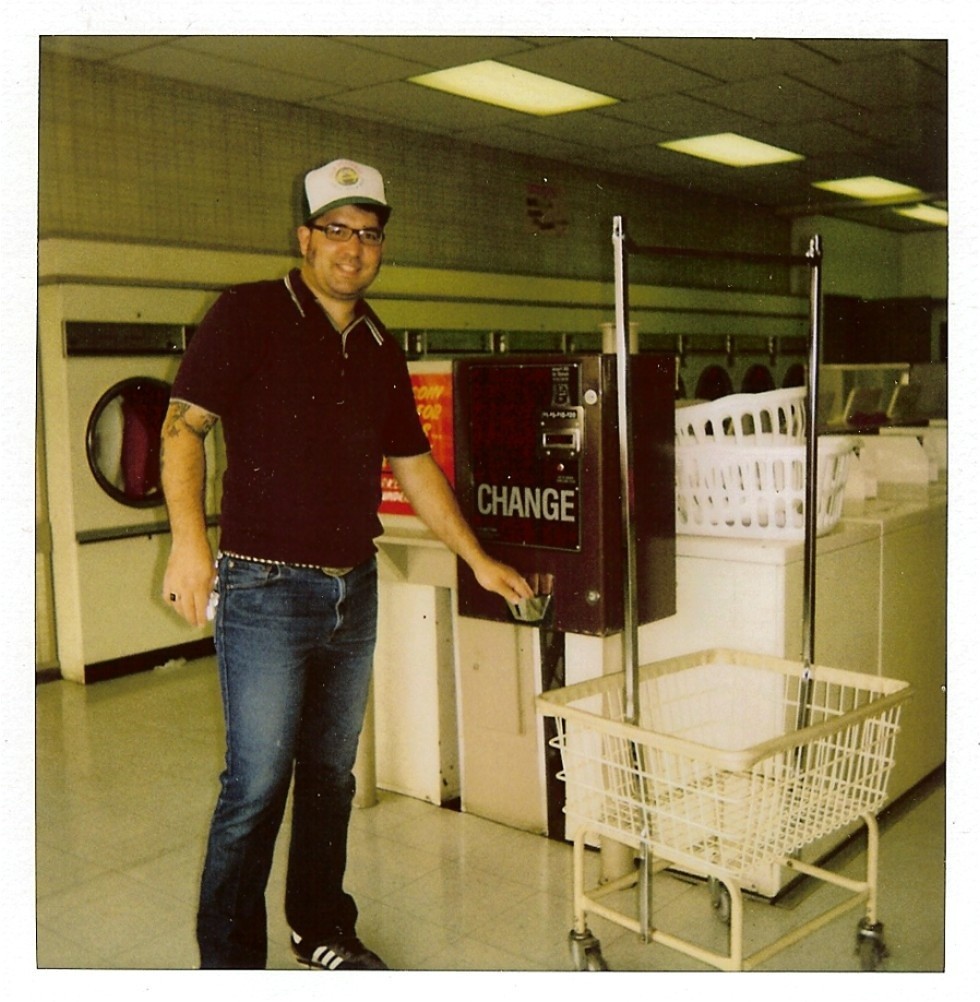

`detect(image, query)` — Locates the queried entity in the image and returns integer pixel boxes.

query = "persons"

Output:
[157,162,539,970]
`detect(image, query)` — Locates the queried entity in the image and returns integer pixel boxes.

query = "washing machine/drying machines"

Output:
[249,254,811,401]
[35,238,248,685]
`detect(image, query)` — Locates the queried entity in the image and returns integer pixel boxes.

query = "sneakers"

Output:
[291,916,390,971]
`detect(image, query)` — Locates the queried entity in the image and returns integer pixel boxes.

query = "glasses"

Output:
[312,222,386,246]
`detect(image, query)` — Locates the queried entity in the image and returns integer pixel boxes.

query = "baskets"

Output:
[675,383,853,541]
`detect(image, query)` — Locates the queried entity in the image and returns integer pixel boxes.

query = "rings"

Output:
[170,591,180,601]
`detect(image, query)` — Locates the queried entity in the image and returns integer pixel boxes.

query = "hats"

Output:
[300,156,394,232]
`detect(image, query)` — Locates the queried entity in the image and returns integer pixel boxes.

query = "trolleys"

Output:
[530,648,914,971]
